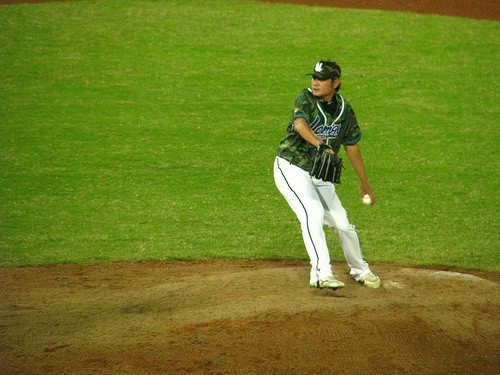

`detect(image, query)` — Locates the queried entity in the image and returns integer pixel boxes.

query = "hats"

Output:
[305,61,341,80]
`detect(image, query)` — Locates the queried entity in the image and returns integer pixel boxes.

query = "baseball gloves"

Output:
[308,143,347,187]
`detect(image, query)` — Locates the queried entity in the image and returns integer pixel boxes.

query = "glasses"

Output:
[313,75,322,80]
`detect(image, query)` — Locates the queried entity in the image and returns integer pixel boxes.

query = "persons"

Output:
[273,61,382,290]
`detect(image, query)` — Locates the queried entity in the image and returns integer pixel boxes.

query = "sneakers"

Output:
[310,278,345,288]
[352,272,381,289]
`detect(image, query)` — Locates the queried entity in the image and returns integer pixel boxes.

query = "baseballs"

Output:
[362,194,372,205]
